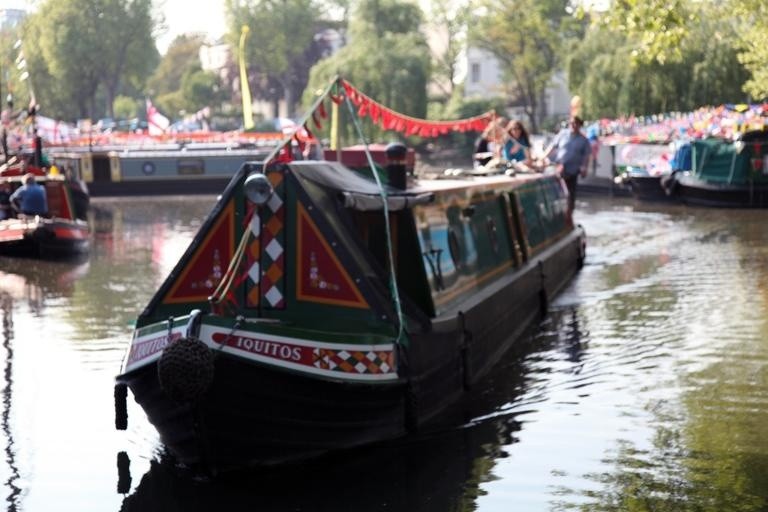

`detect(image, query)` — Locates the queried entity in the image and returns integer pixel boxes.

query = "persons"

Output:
[539,117,591,216]
[499,119,532,168]
[9,173,49,219]
[472,117,507,169]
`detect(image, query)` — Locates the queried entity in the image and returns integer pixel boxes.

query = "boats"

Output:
[0,173,99,258]
[114,78,586,489]
[609,135,768,209]
[14,142,279,198]
